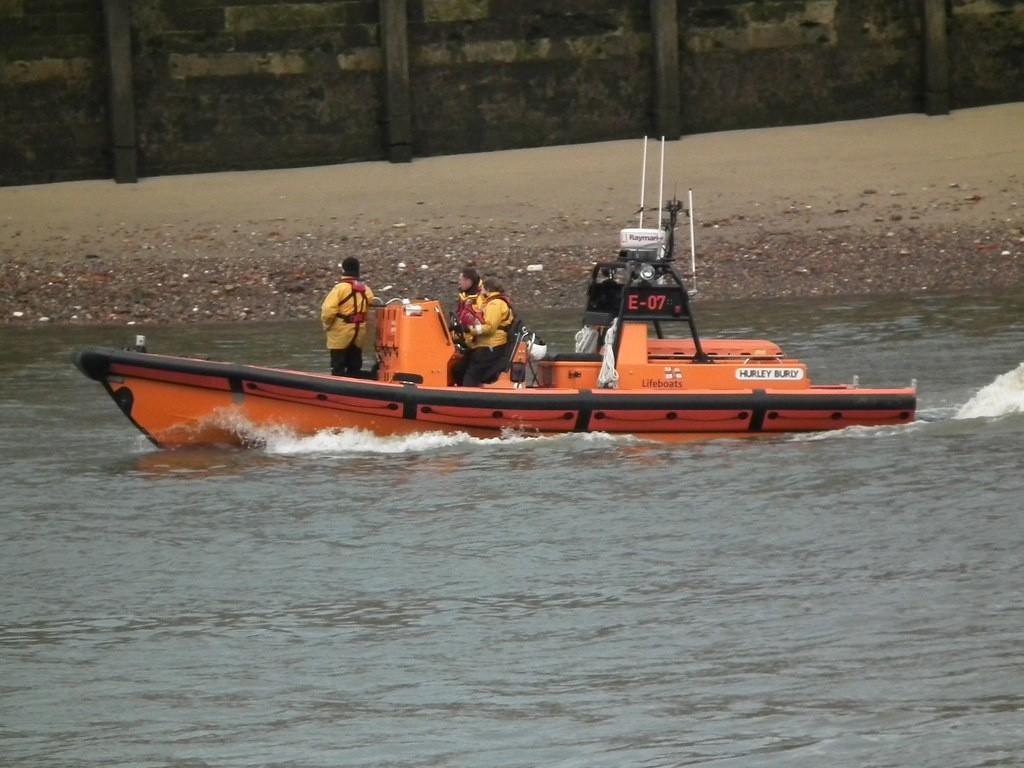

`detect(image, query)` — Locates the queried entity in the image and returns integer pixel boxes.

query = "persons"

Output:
[452,267,516,388]
[319,256,375,380]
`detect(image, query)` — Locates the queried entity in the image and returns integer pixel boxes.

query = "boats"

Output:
[76,134,921,445]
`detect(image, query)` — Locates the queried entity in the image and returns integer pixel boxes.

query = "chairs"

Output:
[479,316,524,383]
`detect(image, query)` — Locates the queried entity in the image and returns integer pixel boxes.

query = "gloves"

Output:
[454,325,468,332]
